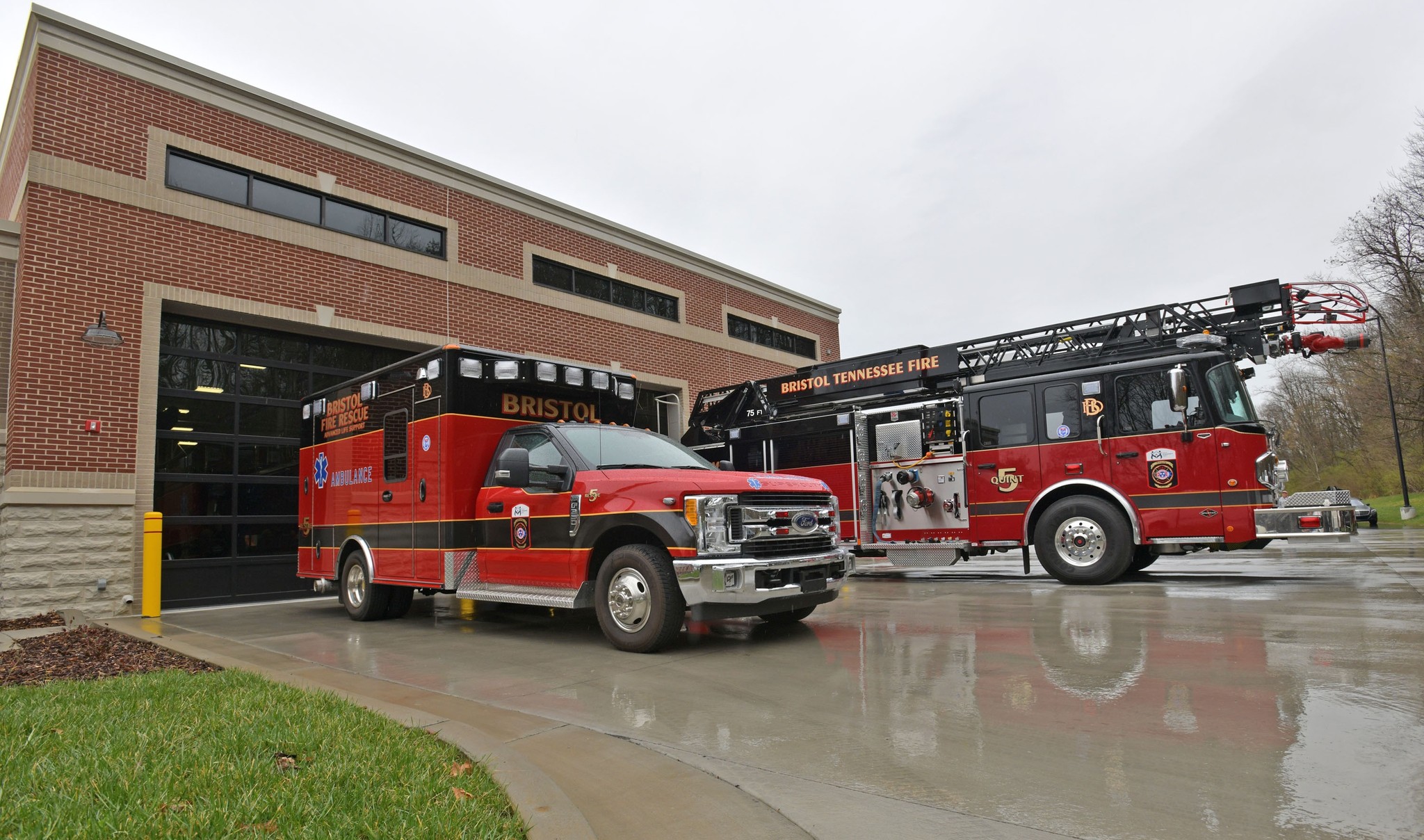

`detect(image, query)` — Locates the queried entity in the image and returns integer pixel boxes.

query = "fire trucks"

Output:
[298,344,856,653]
[678,278,1376,587]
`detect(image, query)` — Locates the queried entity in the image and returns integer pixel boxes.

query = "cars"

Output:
[1351,497,1378,524]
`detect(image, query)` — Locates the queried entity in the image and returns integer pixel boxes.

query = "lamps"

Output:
[81,311,124,350]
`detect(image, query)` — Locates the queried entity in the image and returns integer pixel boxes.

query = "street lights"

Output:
[1350,315,1418,520]
